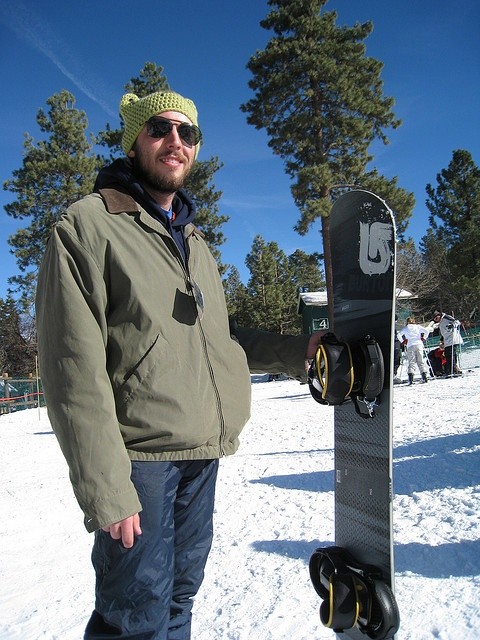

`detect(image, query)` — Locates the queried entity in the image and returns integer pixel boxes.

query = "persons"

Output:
[396,316,429,385]
[37,90,343,640]
[393,314,406,384]
[431,310,462,379]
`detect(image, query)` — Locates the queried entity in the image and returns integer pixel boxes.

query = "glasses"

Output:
[147,116,202,145]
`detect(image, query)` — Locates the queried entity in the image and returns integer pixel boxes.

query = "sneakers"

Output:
[421,378,428,383]
[408,383,415,386]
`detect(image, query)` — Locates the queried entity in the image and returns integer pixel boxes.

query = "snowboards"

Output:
[319,185,399,640]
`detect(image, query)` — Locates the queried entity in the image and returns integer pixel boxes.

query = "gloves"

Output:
[403,339,408,345]
[421,337,425,342]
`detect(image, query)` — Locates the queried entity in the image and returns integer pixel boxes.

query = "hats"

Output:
[120,91,200,161]
[433,310,440,318]
